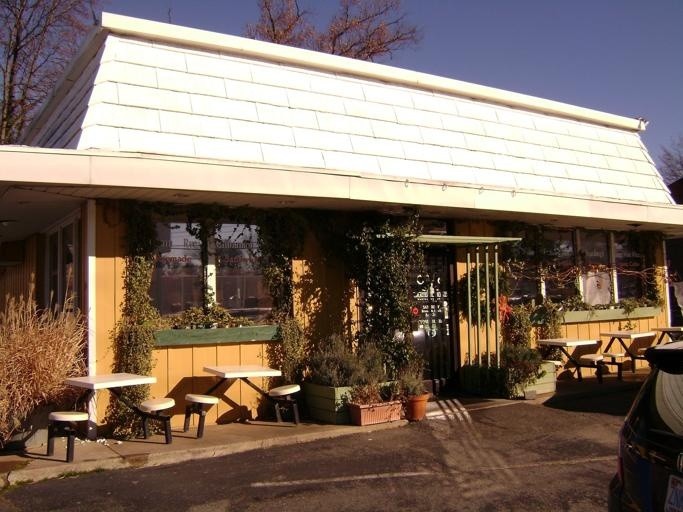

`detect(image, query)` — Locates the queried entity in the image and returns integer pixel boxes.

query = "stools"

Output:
[46,411,90,463]
[538,324,682,387]
[183,394,220,440]
[137,397,177,446]
[267,384,302,426]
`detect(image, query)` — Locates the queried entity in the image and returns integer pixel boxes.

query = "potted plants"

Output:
[400,347,433,422]
[344,375,404,426]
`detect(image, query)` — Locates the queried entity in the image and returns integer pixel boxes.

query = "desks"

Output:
[49,365,176,463]
[182,363,303,440]
